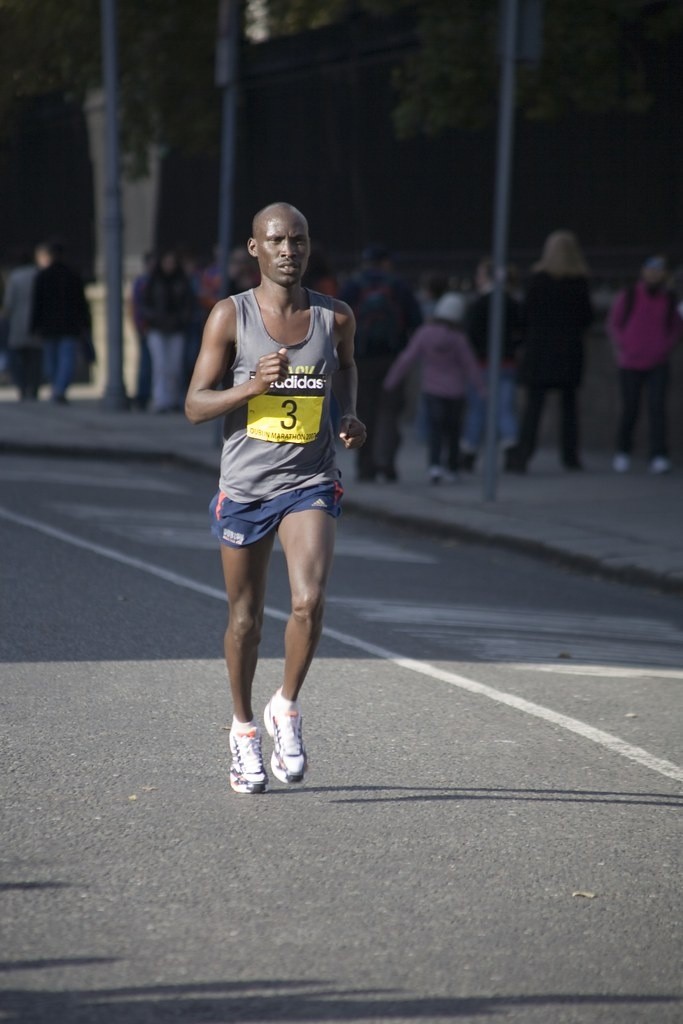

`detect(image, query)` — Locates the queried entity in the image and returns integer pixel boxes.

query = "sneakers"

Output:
[229,724,269,794]
[264,688,307,783]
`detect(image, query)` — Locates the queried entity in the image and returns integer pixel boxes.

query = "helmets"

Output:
[433,293,466,322]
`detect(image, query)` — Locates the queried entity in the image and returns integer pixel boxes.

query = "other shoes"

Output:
[428,466,440,483]
[650,456,670,475]
[444,470,457,483]
[613,453,630,473]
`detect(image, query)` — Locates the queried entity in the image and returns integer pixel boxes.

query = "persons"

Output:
[0,236,90,404]
[185,202,368,794]
[461,252,524,471]
[504,228,597,474]
[134,232,338,415]
[342,243,420,483]
[605,249,683,474]
[385,287,489,485]
